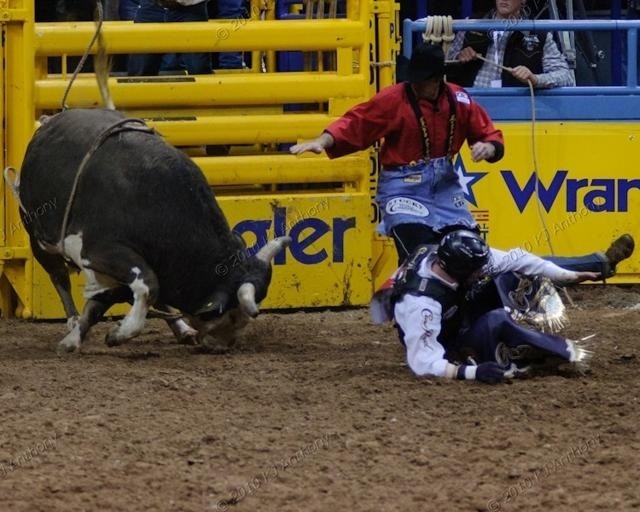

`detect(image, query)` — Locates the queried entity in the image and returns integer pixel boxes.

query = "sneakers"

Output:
[605,234,634,277]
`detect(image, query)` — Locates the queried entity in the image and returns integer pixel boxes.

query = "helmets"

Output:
[437,229,489,284]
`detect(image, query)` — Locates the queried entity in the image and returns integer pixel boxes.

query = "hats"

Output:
[397,44,469,81]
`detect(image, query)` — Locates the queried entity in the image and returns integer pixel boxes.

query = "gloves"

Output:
[457,362,509,386]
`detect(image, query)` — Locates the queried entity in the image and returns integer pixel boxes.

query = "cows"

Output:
[18,107,293,360]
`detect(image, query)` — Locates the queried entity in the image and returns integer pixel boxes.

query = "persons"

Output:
[126,1,232,157]
[290,39,505,266]
[443,3,575,93]
[360,226,636,387]
[219,0,304,151]
